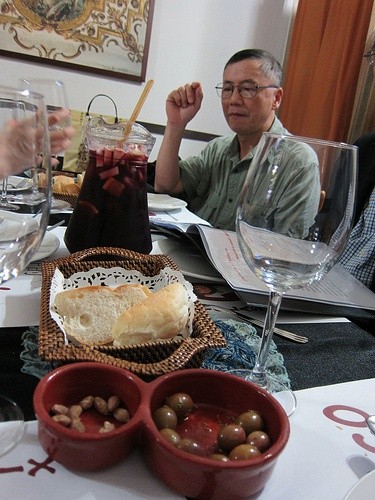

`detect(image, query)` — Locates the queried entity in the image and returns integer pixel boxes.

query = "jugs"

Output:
[64,116,156,255]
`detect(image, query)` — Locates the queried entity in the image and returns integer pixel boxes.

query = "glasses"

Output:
[215,80,280,98]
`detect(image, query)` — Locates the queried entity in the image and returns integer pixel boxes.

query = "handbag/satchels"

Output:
[61,94,129,173]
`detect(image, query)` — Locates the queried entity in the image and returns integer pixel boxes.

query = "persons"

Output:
[0,105,76,182]
[193,282,233,300]
[333,187,375,291]
[154,48,322,240]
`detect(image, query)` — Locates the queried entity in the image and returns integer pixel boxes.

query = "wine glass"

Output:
[224,132,358,417]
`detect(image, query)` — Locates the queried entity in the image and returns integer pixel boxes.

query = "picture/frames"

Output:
[0,0,155,83]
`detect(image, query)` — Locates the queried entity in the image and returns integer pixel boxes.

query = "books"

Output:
[149,219,375,317]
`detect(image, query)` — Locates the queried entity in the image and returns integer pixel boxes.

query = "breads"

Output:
[54,283,151,345]
[112,282,190,346]
[37,172,80,195]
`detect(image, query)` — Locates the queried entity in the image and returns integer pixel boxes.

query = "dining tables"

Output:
[1,173,375,500]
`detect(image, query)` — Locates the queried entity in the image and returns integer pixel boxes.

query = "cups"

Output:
[1,87,53,285]
[14,78,69,156]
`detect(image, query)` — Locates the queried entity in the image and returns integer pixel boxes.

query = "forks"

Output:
[1,178,29,189]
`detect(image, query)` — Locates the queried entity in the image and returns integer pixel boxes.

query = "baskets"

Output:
[37,246,228,375]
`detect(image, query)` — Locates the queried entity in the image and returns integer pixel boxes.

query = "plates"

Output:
[148,192,188,211]
[150,237,227,283]
[0,175,34,192]
[343,471,375,500]
[31,230,60,262]
